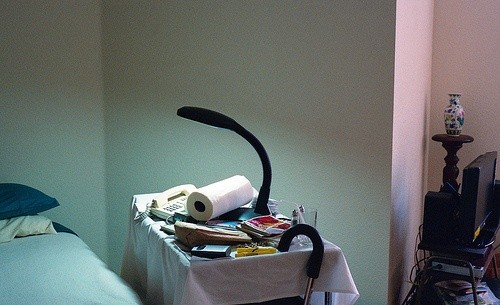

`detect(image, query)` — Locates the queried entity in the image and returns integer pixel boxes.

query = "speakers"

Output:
[492,180,500,226]
[422,189,460,245]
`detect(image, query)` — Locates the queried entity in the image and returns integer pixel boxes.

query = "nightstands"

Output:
[118,192,360,305]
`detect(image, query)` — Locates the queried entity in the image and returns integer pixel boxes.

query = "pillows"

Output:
[0,183,60,220]
[0,213,57,243]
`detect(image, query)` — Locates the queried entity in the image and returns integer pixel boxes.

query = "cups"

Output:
[291,208,318,247]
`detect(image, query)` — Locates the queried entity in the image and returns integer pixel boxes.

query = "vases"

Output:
[444,94,464,136]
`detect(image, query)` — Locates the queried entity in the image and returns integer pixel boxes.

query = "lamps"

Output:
[177,107,272,221]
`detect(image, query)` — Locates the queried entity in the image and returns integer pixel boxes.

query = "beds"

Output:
[0,215,142,305]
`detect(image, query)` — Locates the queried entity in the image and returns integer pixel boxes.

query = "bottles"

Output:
[444,93,464,136]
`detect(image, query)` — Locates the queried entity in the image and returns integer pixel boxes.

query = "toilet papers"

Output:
[186,174,254,222]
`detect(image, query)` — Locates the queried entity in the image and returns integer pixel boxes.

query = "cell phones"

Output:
[191,244,231,257]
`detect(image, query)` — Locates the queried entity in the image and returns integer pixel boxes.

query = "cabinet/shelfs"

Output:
[415,242,499,305]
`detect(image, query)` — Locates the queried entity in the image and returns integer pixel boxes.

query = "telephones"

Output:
[150,183,197,222]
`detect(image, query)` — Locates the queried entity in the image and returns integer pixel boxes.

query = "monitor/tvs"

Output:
[460,149,498,248]
